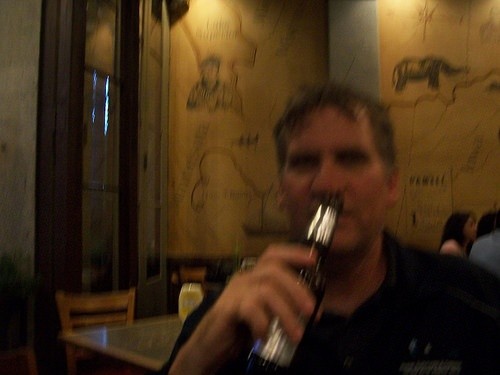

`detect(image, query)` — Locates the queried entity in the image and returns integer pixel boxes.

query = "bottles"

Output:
[244,194,343,375]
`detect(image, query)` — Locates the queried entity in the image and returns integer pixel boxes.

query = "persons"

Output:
[438,211,500,277]
[160,88,500,375]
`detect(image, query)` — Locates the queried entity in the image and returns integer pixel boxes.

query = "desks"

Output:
[58,315,186,375]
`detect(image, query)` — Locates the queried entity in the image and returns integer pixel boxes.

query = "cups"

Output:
[178,283,205,323]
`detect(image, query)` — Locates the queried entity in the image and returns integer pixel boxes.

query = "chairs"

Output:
[58,286,135,375]
